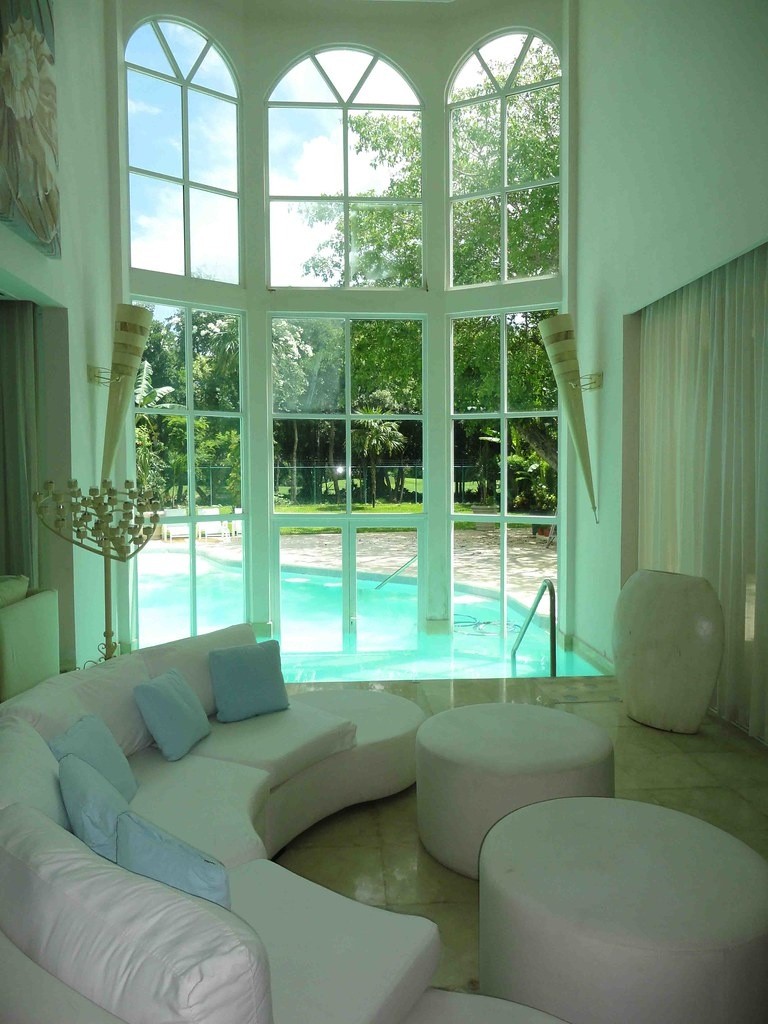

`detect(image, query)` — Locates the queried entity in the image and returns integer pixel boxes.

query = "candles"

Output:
[34,479,160,555]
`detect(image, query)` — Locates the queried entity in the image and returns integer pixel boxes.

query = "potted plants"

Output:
[471,444,499,531]
[516,469,556,535]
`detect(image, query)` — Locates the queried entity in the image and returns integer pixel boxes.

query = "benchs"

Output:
[162,508,242,542]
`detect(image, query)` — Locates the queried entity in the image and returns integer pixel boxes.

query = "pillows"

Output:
[209,640,290,722]
[56,754,129,862]
[47,713,138,804]
[114,811,232,911]
[132,665,211,762]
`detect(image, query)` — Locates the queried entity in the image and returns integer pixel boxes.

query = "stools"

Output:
[479,797,768,1024]
[417,702,616,881]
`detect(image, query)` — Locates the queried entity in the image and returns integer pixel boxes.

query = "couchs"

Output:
[0,624,440,1024]
[0,573,59,702]
